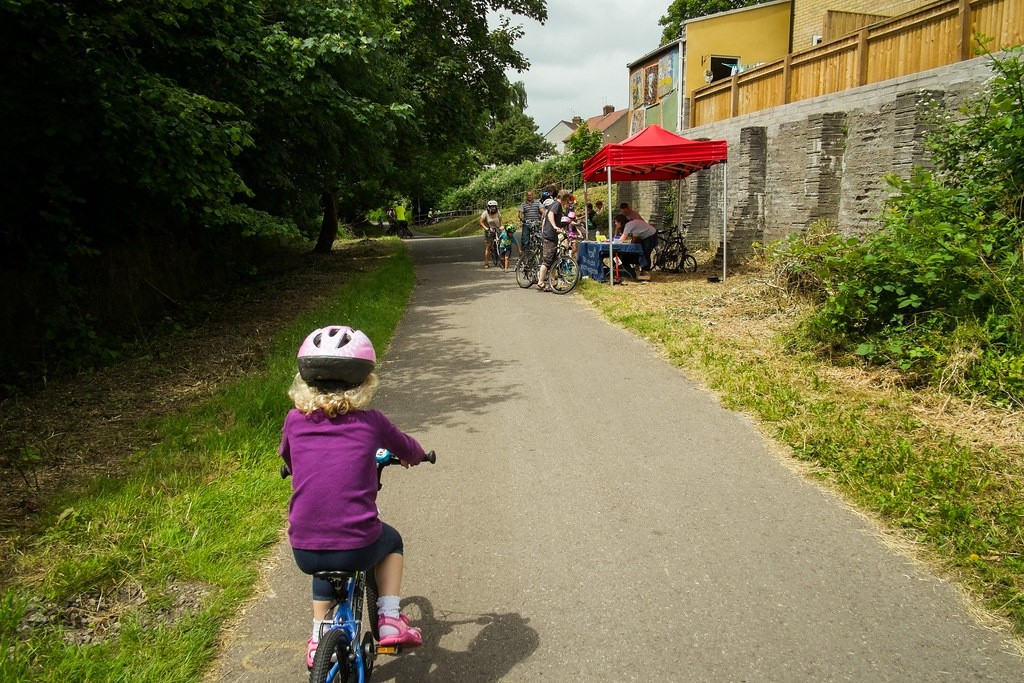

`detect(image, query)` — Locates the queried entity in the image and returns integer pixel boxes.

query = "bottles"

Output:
[596,230,600,238]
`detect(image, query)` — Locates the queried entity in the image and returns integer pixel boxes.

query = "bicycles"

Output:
[278,447,437,683]
[514,217,582,295]
[650,224,697,273]
[377,222,414,238]
[485,228,504,267]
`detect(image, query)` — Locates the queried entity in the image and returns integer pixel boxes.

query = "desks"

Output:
[580,241,642,283]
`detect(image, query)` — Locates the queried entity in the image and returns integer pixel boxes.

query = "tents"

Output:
[583,124,728,287]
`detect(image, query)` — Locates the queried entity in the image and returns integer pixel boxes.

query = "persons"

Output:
[480,189,657,292]
[377,216,383,224]
[278,325,424,668]
[426,208,433,226]
[388,208,395,217]
[394,202,406,222]
[434,210,442,222]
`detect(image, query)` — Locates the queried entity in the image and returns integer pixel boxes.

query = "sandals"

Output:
[377,613,423,646]
[553,284,565,292]
[536,283,552,292]
[306,636,320,672]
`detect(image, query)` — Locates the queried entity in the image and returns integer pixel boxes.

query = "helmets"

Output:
[504,224,517,233]
[487,201,498,214]
[296,325,376,385]
[568,212,576,219]
[542,192,551,202]
[560,216,571,223]
